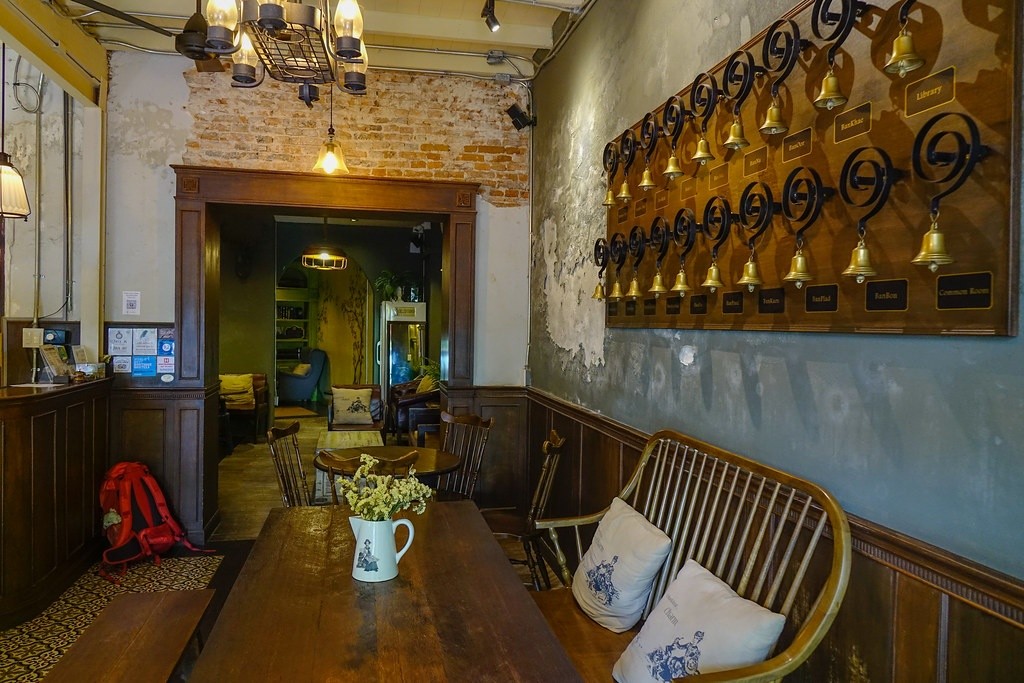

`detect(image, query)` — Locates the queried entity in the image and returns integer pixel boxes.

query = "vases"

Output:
[349,517,415,582]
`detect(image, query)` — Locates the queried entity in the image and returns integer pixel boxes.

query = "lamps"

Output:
[301,216,349,271]
[505,103,538,131]
[481,0,500,33]
[207,0,373,111]
[313,23,348,177]
[0,151,31,222]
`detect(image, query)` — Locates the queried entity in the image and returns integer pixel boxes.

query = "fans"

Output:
[70,0,225,72]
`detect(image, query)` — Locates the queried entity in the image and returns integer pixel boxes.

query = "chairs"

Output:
[482,430,567,592]
[391,380,438,446]
[313,446,461,507]
[409,407,441,448]
[327,384,390,446]
[432,410,494,504]
[320,450,419,505]
[223,373,268,443]
[267,420,311,508]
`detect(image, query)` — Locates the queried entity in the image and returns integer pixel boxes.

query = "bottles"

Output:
[411,282,418,302]
[280,306,293,319]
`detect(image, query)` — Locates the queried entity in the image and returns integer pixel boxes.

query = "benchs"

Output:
[41,588,224,683]
[527,427,851,683]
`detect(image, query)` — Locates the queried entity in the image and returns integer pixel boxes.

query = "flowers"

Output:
[336,453,436,521]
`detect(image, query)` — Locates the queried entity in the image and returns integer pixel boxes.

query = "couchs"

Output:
[277,347,324,400]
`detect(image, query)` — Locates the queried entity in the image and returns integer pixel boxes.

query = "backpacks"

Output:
[98,461,217,586]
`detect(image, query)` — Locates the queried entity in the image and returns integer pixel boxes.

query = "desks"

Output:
[187,502,589,683]
[315,431,383,507]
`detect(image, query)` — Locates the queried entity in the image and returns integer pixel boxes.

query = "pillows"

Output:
[612,559,786,683]
[416,374,436,394]
[293,364,311,376]
[331,387,373,424]
[219,374,255,410]
[572,498,671,633]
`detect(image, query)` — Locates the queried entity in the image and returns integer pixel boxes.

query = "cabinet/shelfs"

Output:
[0,375,113,632]
[276,287,319,403]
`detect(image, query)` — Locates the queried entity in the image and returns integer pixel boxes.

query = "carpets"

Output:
[0,539,256,683]
[275,405,323,419]
